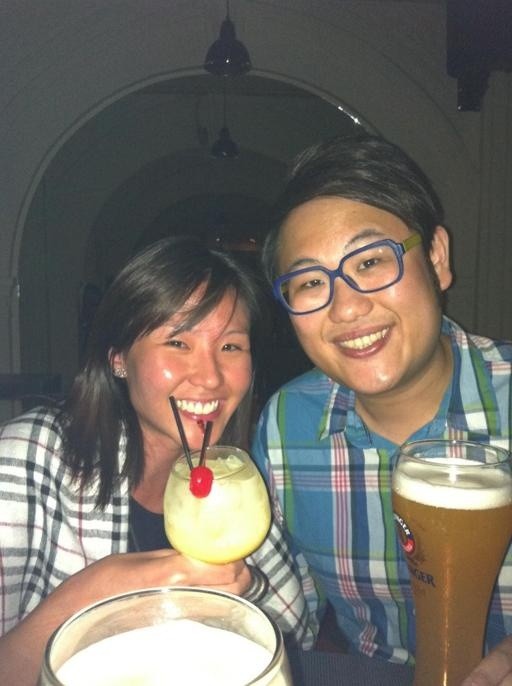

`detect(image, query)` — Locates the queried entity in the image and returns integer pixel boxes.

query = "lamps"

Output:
[204,0,253,80]
[209,92,241,162]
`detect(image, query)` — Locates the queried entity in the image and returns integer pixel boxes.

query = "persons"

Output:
[0,229,328,686]
[241,129,512,686]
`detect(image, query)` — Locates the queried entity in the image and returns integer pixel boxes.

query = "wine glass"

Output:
[162,445,271,605]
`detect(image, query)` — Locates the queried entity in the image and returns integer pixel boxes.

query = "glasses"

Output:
[274,233,421,315]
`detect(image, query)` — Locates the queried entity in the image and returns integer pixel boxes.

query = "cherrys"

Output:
[190,466,212,498]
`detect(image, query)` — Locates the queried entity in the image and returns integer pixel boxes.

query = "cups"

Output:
[388,437,512,686]
[37,583,295,686]
